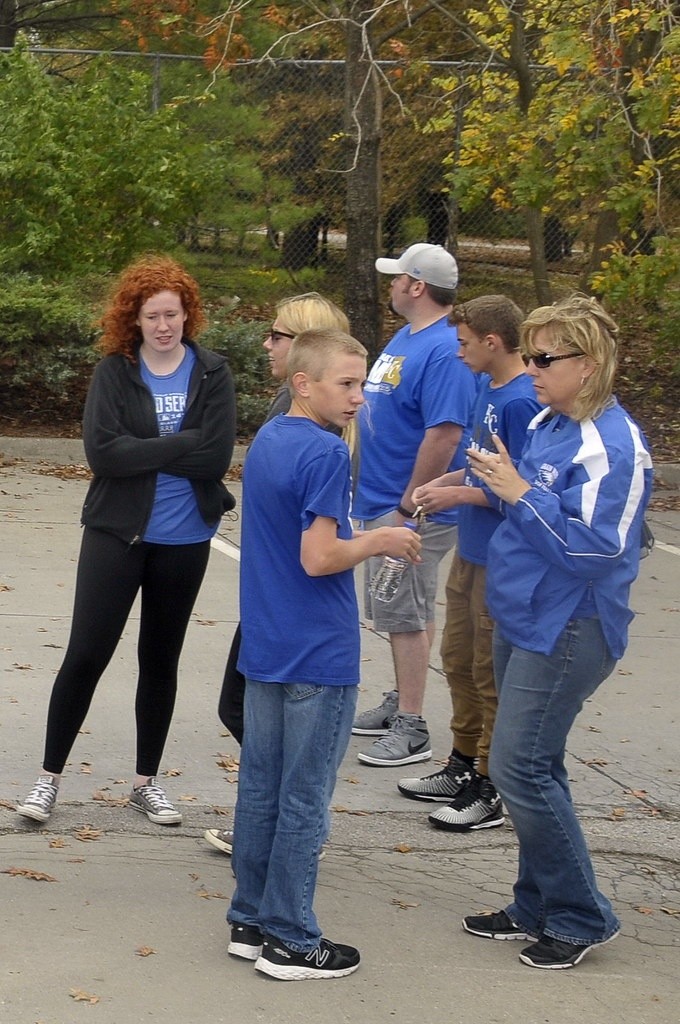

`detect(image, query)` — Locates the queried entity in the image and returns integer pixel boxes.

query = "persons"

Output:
[16,259,235,823]
[543,203,572,271]
[460,298,654,969]
[621,211,673,276]
[351,244,478,766]
[397,294,546,833]
[205,293,422,980]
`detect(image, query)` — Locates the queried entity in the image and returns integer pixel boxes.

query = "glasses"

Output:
[270,328,294,341]
[521,352,586,368]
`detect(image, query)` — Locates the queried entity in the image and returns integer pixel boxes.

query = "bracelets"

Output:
[397,504,420,520]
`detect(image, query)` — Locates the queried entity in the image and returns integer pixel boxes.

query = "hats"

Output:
[375,243,458,289]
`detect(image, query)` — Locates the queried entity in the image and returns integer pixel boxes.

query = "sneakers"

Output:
[317,846,326,860]
[462,910,539,943]
[227,921,263,960]
[357,711,432,766]
[204,828,234,854]
[19,774,59,822]
[428,772,505,832]
[128,776,183,824]
[351,690,399,735]
[398,755,475,802]
[519,922,623,970]
[254,934,360,980]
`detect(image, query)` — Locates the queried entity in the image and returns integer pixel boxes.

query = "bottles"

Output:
[368,520,417,604]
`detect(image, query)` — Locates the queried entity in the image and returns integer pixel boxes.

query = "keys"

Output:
[412,505,423,517]
[419,512,425,523]
[411,503,429,529]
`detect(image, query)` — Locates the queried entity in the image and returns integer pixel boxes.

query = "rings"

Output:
[485,469,493,475]
